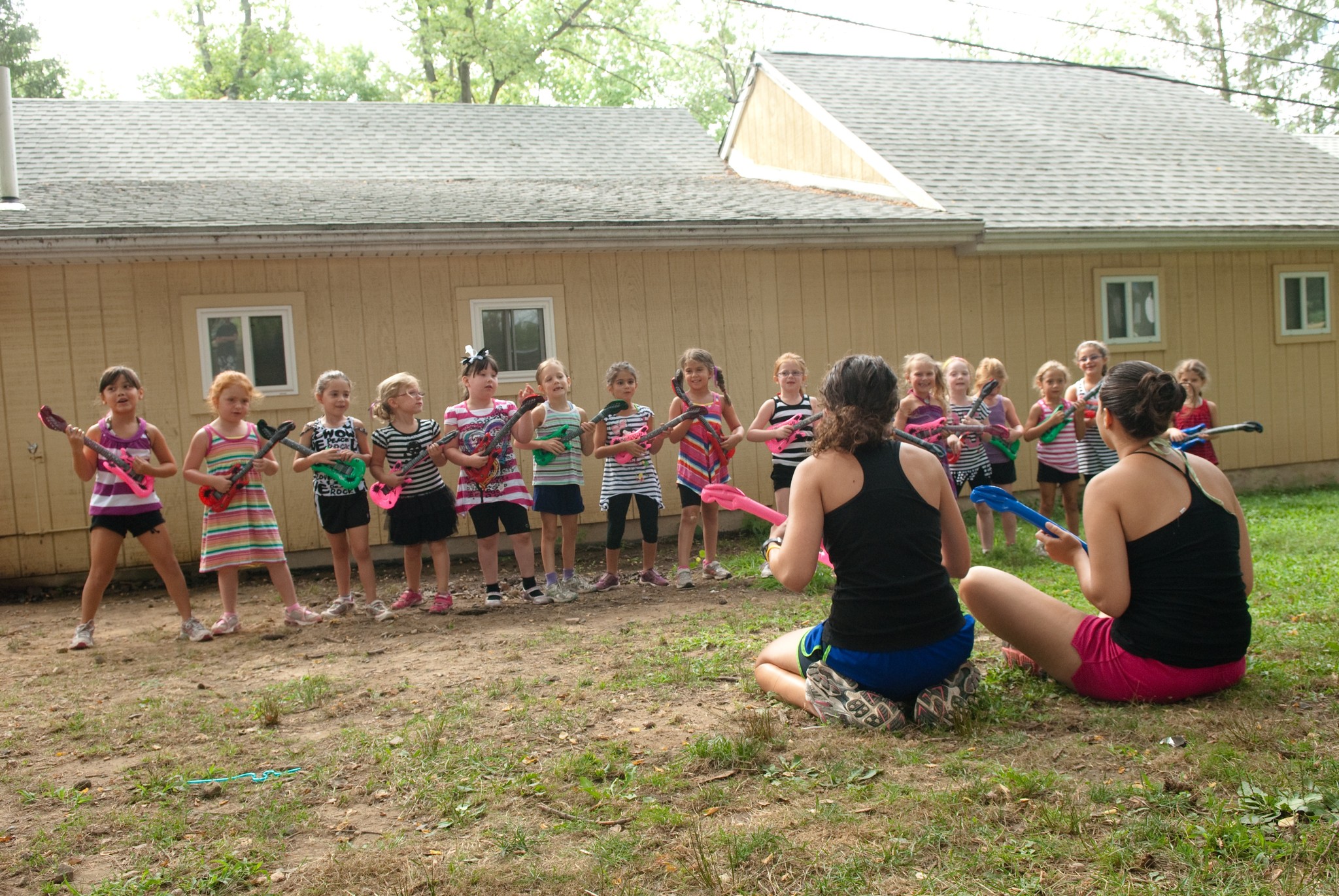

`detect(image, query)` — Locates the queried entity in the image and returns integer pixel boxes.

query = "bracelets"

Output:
[763,545,783,563]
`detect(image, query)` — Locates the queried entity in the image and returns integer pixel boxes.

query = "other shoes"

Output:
[1035,540,1050,557]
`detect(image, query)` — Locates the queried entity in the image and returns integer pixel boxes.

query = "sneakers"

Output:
[674,565,695,588]
[283,604,322,626]
[522,585,551,604]
[701,559,732,580]
[390,586,424,610]
[592,571,620,592]
[68,623,96,649]
[364,601,395,622]
[544,581,578,603]
[319,594,359,620]
[803,659,908,734]
[210,611,241,635]
[561,575,597,594]
[428,591,453,614]
[484,591,503,608]
[912,659,981,731]
[639,568,670,587]
[1001,637,1048,678]
[760,561,774,578]
[178,616,213,642]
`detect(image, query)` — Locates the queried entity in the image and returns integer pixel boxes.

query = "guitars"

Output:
[968,483,1094,557]
[946,379,999,463]
[902,418,1011,443]
[531,399,629,467]
[1169,421,1264,449]
[670,369,736,468]
[610,405,707,465]
[464,394,545,483]
[256,418,366,490]
[1034,376,1104,444]
[368,430,459,509]
[699,483,835,574]
[988,430,1021,458]
[197,421,296,514]
[763,406,828,455]
[36,404,155,498]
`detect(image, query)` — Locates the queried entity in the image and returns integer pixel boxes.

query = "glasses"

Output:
[1077,354,1104,361]
[393,389,425,399]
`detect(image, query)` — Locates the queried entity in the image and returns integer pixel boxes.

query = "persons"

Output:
[590,360,675,593]
[1160,360,1223,470]
[181,368,325,637]
[887,352,961,502]
[1021,357,1087,557]
[953,358,1255,706]
[751,354,982,729]
[213,318,238,371]
[746,352,834,581]
[940,356,997,554]
[62,363,214,649]
[668,348,746,591]
[1066,336,1119,489]
[514,356,599,605]
[438,345,550,608]
[973,358,1026,551]
[370,372,459,616]
[292,368,399,626]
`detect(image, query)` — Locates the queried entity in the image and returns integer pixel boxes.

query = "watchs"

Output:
[756,537,784,561]
